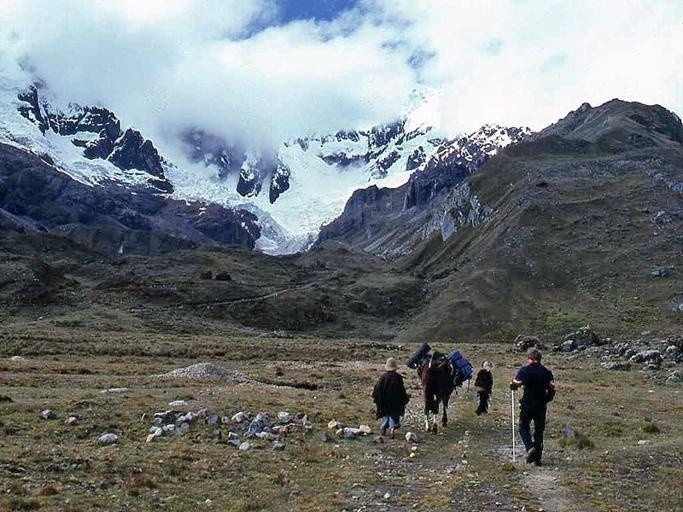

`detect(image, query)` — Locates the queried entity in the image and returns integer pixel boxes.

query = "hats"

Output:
[384,357,399,372]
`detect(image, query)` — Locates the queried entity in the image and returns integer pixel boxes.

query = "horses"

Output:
[417,358,456,434]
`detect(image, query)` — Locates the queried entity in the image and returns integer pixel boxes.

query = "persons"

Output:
[509,347,556,466]
[474,360,493,416]
[371,357,409,439]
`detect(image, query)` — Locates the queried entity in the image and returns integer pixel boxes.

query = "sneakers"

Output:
[527,448,537,462]
